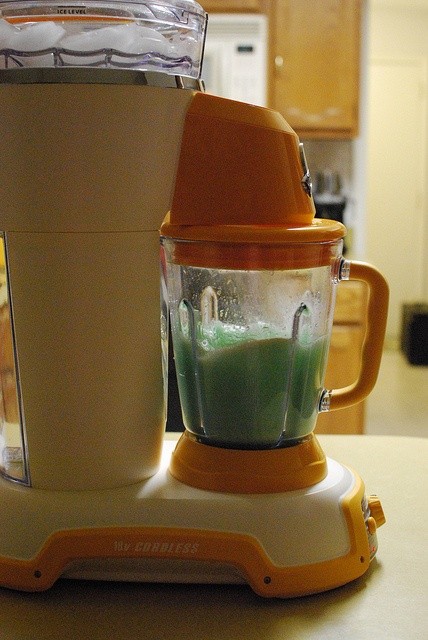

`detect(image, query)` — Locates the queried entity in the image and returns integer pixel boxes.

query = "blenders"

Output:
[0,1,389,598]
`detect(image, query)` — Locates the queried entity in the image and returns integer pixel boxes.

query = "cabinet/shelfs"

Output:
[266,0,362,140]
